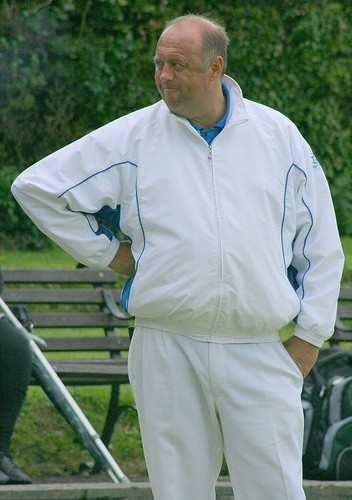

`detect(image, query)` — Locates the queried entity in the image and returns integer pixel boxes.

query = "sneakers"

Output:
[1,456,33,483]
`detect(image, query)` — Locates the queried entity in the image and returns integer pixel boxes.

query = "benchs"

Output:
[0,268,314,475]
[310,286,352,481]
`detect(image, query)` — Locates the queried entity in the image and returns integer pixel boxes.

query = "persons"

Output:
[9,15,347,500]
